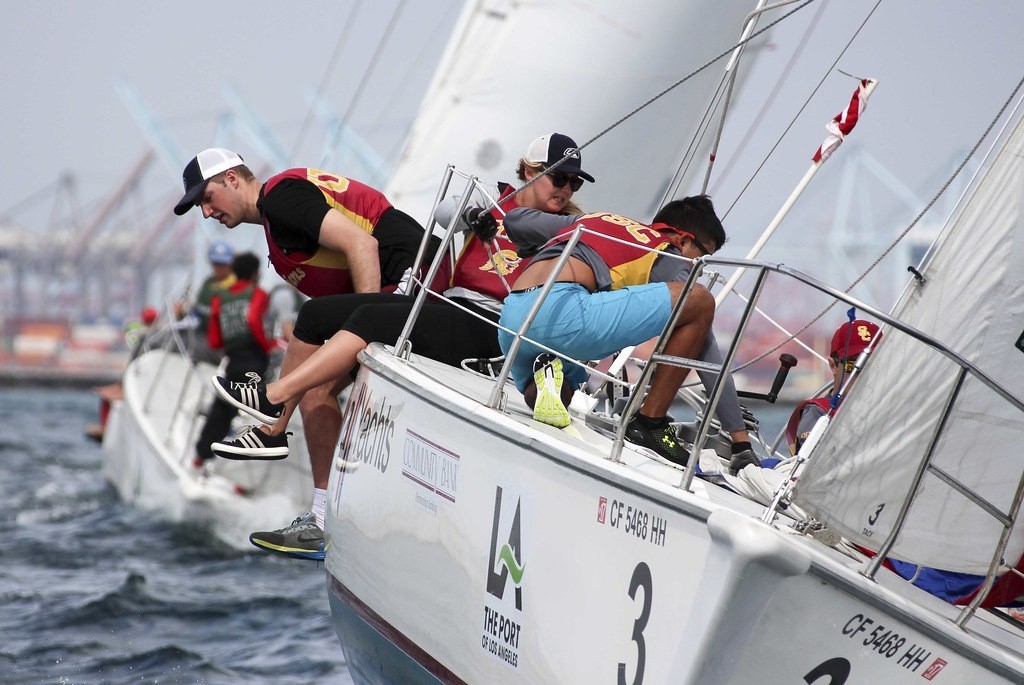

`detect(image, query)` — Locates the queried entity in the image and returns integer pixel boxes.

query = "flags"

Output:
[812,79,868,163]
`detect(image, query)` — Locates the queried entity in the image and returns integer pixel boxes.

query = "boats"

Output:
[97,265,327,561]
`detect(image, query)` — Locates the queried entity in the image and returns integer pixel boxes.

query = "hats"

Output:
[141,308,157,324]
[173,149,244,216]
[830,320,883,360]
[209,241,233,264]
[527,133,595,183]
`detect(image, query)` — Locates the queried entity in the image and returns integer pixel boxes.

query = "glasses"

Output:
[833,357,866,374]
[537,166,584,192]
[691,237,712,266]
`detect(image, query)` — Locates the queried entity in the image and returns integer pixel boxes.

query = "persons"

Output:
[784,320,883,458]
[211,132,595,462]
[85,240,307,472]
[498,194,760,477]
[174,148,457,563]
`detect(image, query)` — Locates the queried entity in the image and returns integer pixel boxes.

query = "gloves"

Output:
[469,207,498,242]
[729,449,763,476]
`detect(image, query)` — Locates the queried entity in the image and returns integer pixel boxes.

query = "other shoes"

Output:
[191,456,208,475]
[235,478,253,500]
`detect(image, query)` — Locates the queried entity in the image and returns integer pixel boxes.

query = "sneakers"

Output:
[623,410,690,470]
[210,423,294,460]
[249,510,327,561]
[211,372,286,425]
[533,353,571,429]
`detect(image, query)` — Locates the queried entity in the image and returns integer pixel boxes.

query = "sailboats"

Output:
[316,0,1024,684]
